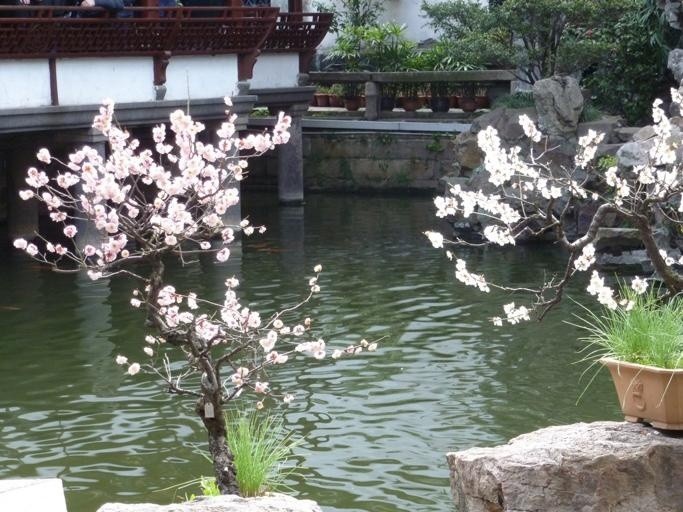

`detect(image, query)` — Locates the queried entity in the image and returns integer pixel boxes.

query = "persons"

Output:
[64,0,125,18]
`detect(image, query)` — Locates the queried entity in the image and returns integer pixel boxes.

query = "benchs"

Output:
[0,0,333,58]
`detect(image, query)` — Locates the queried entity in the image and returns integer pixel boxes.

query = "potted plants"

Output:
[311,22,488,113]
[557,284,683,436]
